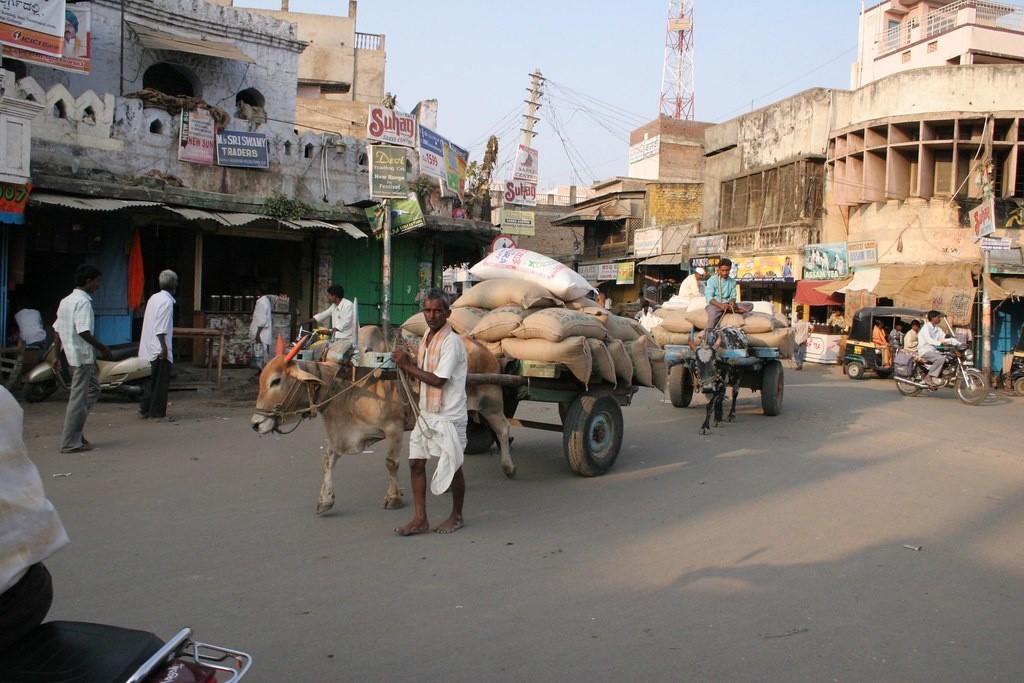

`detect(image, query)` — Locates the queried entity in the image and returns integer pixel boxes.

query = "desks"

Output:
[8,344,40,373]
[168,328,224,390]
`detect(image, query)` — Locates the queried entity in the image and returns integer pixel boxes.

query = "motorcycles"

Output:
[288,326,335,350]
[842,306,957,380]
[1,618,253,683]
[892,334,988,406]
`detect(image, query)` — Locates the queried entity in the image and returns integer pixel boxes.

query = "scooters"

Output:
[23,333,151,402]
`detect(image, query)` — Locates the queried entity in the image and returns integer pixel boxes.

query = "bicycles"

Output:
[1000,346,1017,389]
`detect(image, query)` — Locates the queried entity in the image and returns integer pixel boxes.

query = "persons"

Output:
[808,249,844,276]
[792,306,846,370]
[64,9,81,54]
[679,266,705,297]
[872,317,921,368]
[918,310,952,387]
[249,287,272,370]
[297,284,361,340]
[698,258,753,339]
[634,291,653,322]
[132,269,178,422]
[390,288,468,535]
[0,383,72,654]
[51,264,113,454]
[782,256,792,276]
[14,293,47,346]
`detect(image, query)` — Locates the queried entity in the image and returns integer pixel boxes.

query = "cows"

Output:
[686,324,748,436]
[251,332,517,515]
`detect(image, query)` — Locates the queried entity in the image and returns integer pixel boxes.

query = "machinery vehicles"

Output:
[1003,352,1024,396]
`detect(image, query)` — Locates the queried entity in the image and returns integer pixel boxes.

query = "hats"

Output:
[895,321,905,326]
[876,318,883,325]
[911,320,921,327]
[695,267,704,274]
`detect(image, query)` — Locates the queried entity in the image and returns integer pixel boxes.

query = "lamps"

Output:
[251,116,347,154]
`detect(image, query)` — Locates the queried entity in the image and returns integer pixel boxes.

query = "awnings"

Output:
[28,193,368,240]
[126,20,259,64]
[637,255,682,265]
[550,199,632,226]
[795,280,845,305]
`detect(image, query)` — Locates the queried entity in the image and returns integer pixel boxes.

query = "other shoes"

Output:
[795,364,802,370]
[883,362,891,366]
[147,416,175,422]
[923,375,937,387]
